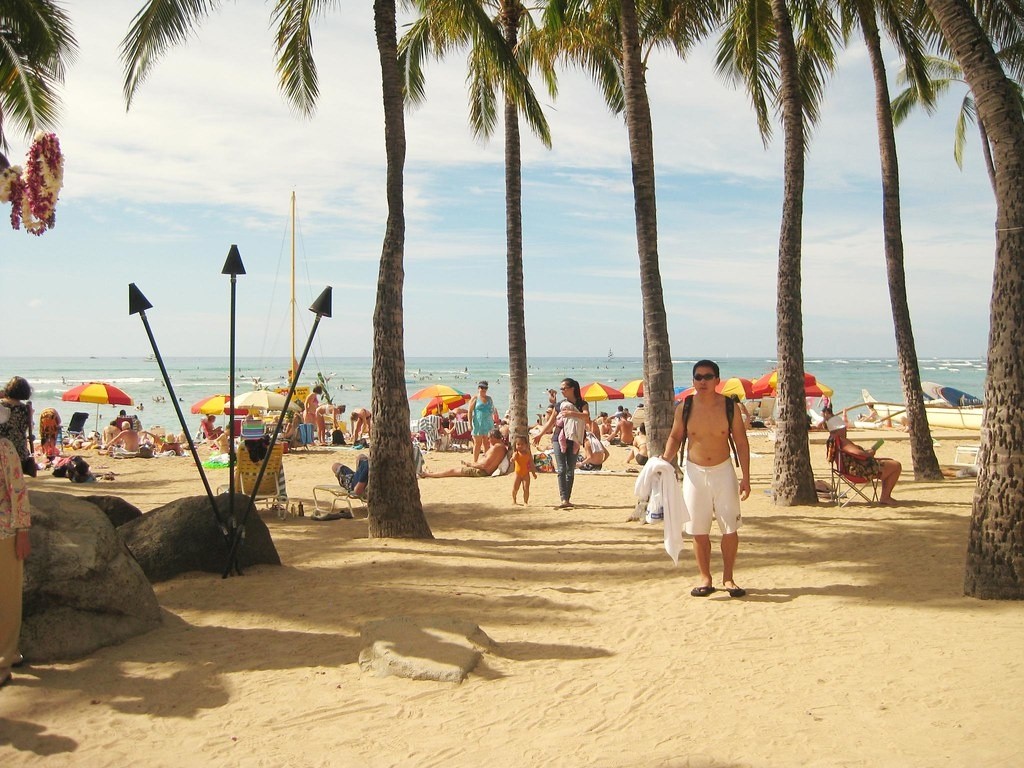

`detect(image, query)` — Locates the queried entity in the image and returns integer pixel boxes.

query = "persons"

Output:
[303,385,372,443]
[136,379,185,410]
[199,413,369,502]
[35,408,62,456]
[98,410,184,456]
[805,393,902,505]
[66,454,97,483]
[62,375,117,418]
[656,359,750,598]
[0,376,36,686]
[179,366,357,391]
[417,381,648,507]
[412,365,630,385]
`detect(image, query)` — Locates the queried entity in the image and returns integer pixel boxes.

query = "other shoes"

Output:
[0,667,11,688]
[10,653,24,667]
[316,513,340,521]
[338,510,353,518]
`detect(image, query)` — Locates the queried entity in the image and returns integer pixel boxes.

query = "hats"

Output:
[0,403,11,423]
[165,433,176,443]
[827,416,847,431]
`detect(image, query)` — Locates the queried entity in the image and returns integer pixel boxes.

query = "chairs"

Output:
[56,412,472,520]
[630,396,883,508]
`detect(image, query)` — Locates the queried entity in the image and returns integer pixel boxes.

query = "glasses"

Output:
[560,387,572,390]
[693,374,716,380]
[479,386,488,389]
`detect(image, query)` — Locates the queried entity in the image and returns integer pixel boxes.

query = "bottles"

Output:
[291,504,296,514]
[297,501,304,516]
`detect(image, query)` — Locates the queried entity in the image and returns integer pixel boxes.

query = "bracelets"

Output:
[17,529,30,532]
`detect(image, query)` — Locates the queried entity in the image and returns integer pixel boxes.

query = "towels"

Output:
[585,431,604,453]
[419,415,440,451]
[634,457,692,566]
[298,424,314,446]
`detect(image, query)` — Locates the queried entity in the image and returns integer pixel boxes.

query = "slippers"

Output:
[724,578,746,597]
[270,504,285,511]
[691,585,715,597]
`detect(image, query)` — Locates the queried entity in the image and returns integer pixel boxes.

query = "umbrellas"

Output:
[675,369,833,400]
[62,382,134,432]
[190,394,248,431]
[580,379,644,417]
[407,385,471,417]
[224,390,302,412]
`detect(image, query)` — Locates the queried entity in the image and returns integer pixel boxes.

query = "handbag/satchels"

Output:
[646,472,664,524]
[22,457,37,477]
